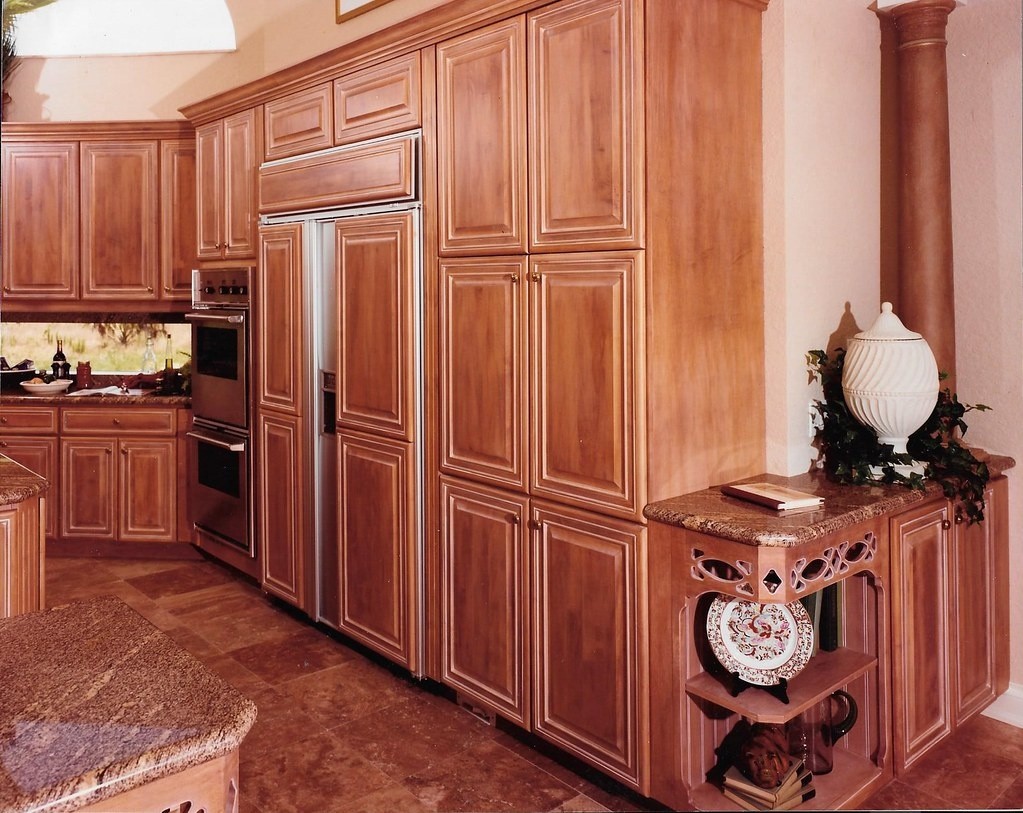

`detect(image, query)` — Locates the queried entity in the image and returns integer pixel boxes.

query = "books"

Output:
[723,755,816,812]
[721,482,825,511]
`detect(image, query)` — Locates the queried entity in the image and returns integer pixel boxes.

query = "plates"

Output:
[706,581,814,688]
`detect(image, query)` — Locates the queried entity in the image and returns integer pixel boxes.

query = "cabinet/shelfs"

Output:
[0,0,1013,812]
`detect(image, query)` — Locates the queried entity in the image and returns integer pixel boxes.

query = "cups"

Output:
[787,688,857,774]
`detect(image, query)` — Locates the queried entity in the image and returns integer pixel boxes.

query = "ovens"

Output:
[184,266,258,560]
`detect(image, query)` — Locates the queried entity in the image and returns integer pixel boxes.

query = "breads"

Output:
[30,377,44,384]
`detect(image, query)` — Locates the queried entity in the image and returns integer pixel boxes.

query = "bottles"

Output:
[143,336,157,375]
[53,340,67,379]
[165,334,173,370]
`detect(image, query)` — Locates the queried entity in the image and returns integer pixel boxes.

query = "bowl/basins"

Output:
[21,379,72,394]
